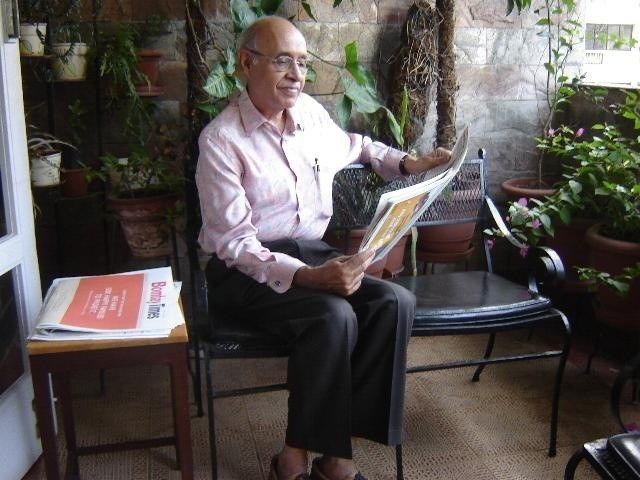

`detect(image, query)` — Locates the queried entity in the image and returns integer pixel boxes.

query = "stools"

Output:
[564,429,640,479]
[23,280,190,480]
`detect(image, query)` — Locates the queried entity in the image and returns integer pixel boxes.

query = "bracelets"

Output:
[399,154,407,176]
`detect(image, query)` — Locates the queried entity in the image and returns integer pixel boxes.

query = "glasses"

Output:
[243,46,312,73]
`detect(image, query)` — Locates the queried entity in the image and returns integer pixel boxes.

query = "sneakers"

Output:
[271,454,367,479]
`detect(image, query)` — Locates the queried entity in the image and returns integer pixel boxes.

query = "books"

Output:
[25,265,184,341]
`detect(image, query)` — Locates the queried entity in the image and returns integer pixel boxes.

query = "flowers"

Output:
[486,111,640,258]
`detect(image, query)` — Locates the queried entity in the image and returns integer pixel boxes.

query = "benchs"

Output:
[181,144,576,480]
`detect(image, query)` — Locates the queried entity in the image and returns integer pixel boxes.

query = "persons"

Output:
[194,15,453,479]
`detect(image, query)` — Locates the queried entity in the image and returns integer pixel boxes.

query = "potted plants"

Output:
[13,2,187,259]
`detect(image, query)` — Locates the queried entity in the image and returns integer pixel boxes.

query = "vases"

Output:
[322,227,392,278]
[385,226,414,279]
[583,218,640,331]
[499,172,561,206]
[411,197,484,261]
